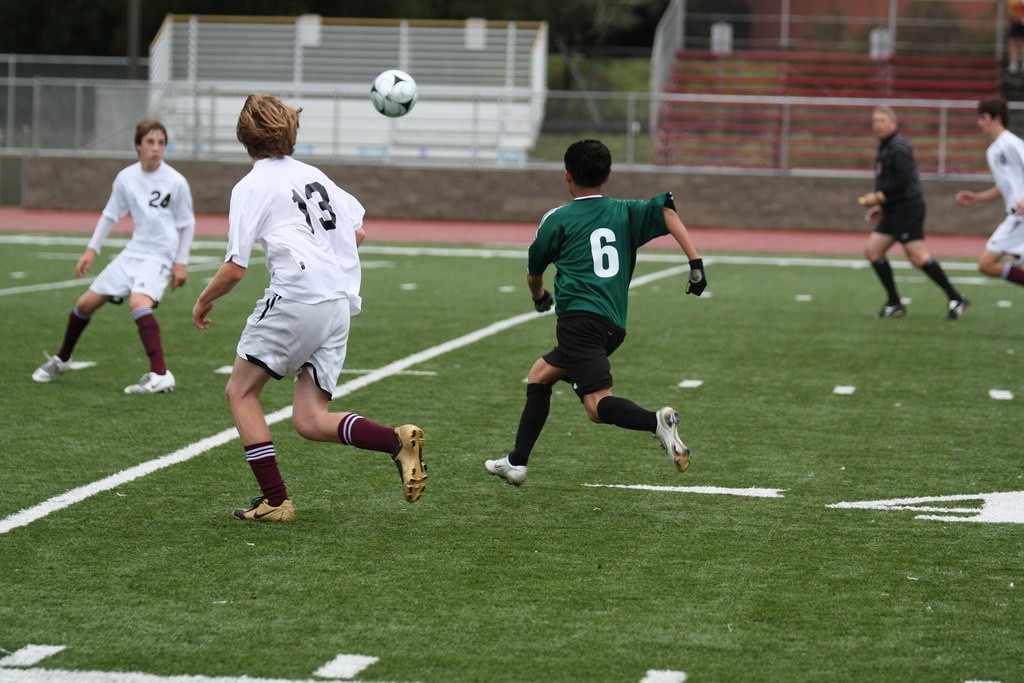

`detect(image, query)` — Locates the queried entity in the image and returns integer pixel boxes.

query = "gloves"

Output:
[686,257,707,297]
[532,290,553,313]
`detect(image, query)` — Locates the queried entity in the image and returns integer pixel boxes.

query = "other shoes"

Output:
[946,296,968,321]
[873,305,906,320]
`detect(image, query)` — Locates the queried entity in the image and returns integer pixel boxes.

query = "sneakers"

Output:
[124,369,175,395]
[32,350,72,382]
[390,423,429,502]
[484,453,526,488]
[232,495,295,524]
[656,406,690,473]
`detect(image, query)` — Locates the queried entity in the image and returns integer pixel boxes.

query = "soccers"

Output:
[369,68,421,119]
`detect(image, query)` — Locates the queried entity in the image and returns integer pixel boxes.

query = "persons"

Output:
[483,138,708,485]
[30,119,196,395]
[956,93,1024,285]
[191,94,427,522]
[1005,0,1024,74]
[856,104,968,320]
